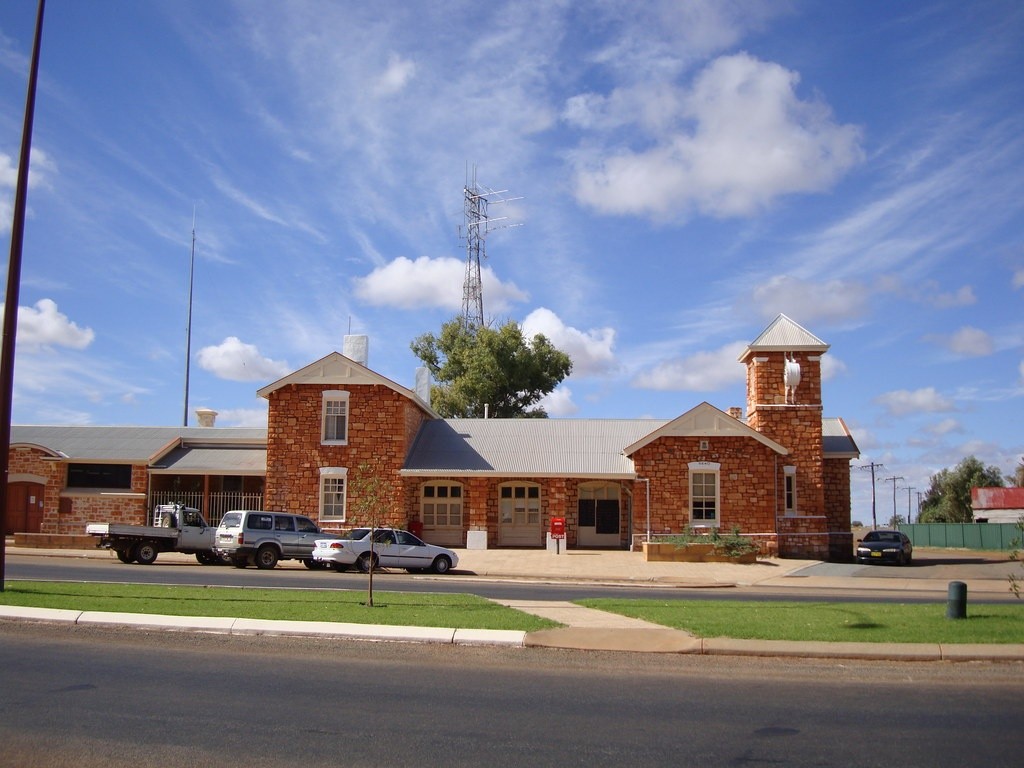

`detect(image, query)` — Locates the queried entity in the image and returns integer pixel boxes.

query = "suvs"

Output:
[211,510,342,570]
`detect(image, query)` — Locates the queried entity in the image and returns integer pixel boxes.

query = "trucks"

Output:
[86,500,217,565]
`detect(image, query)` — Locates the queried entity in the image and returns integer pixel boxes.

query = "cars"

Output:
[312,528,459,574]
[857,530,913,566]
